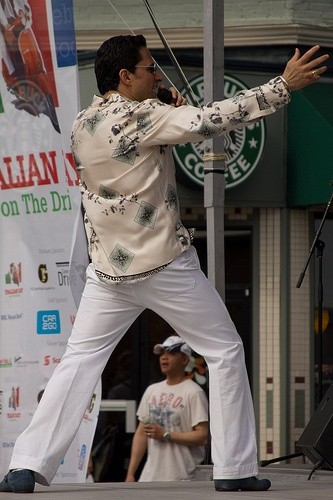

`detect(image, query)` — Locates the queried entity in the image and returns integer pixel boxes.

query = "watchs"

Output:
[160,430,170,443]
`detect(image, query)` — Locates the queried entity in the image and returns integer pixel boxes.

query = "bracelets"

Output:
[279,75,292,94]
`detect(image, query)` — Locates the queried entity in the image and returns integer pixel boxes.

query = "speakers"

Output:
[296,383,333,471]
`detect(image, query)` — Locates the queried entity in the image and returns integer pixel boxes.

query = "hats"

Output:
[154,336,191,359]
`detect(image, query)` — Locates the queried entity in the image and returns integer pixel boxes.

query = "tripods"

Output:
[260,194,333,467]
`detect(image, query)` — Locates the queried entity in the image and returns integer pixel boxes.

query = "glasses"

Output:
[134,63,157,75]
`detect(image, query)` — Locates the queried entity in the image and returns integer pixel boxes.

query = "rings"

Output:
[312,69,318,76]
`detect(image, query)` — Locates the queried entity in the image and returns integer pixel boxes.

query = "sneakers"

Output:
[0,469,35,493]
[214,476,271,492]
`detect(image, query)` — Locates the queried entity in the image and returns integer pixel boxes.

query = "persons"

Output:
[0,33,330,494]
[124,335,210,482]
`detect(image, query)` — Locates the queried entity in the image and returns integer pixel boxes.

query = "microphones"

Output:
[157,88,181,108]
